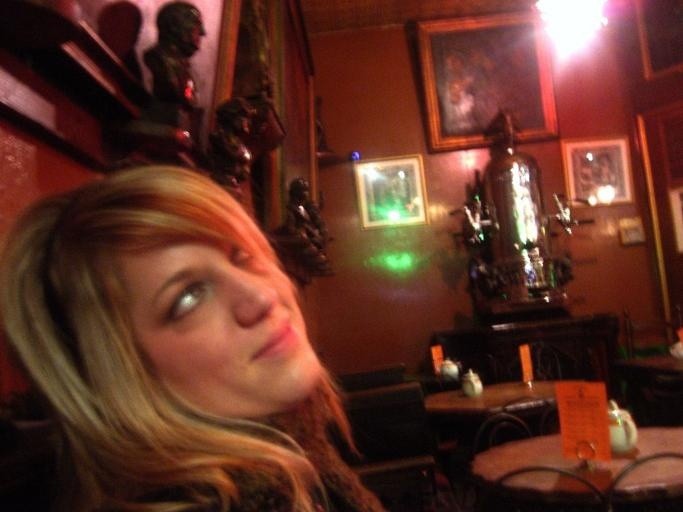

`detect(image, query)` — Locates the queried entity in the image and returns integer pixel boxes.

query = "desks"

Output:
[429,313,622,435]
[424,378,607,511]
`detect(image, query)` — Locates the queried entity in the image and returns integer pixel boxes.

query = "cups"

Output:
[461,368,483,398]
[608,399,638,455]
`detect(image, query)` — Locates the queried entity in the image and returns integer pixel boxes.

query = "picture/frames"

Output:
[634,98,683,346]
[209,0,321,234]
[352,153,430,232]
[634,0,683,81]
[559,135,636,210]
[416,9,561,153]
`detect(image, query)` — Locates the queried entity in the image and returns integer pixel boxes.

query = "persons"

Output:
[142,0,209,112]
[440,39,543,134]
[283,174,335,278]
[205,91,255,188]
[1,162,385,512]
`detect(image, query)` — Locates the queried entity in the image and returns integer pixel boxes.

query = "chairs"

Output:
[459,398,683,512]
